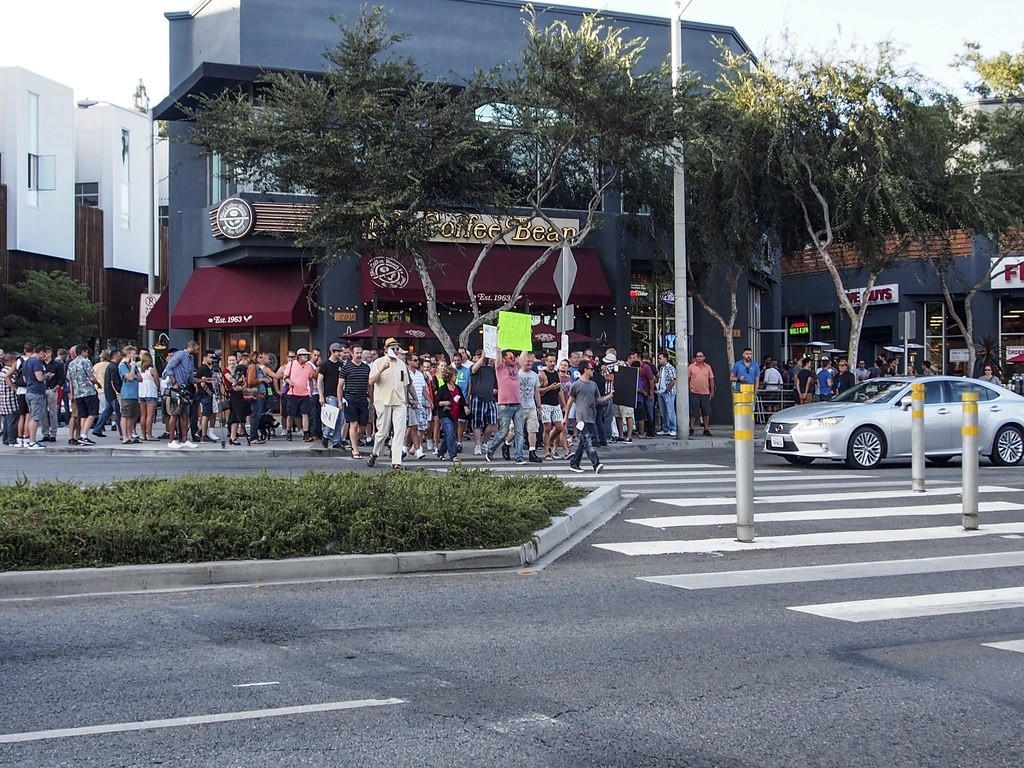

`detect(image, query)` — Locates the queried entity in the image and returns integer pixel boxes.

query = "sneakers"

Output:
[286,432,293,441]
[304,435,315,442]
[367,453,378,467]
[414,448,426,459]
[391,464,404,471]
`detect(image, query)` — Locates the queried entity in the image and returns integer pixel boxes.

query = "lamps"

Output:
[344,326,357,344]
[154,332,169,349]
[597,331,610,347]
[238,333,246,350]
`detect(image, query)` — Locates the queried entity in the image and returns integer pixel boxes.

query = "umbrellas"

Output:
[1005,353,1024,362]
[336,321,460,341]
[531,322,598,344]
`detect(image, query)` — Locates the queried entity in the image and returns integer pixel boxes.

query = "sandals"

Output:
[350,448,362,459]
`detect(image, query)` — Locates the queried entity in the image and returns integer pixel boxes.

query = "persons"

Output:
[922,360,934,375]
[760,353,917,421]
[688,351,715,436]
[561,360,614,474]
[729,347,760,392]
[979,366,1000,400]
[0,324,677,470]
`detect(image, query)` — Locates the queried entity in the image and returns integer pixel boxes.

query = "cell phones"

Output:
[557,383,562,386]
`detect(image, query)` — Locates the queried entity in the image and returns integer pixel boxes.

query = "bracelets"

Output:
[737,378,740,381]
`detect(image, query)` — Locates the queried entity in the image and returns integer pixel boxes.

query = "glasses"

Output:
[504,356,516,360]
[412,359,420,362]
[658,358,665,359]
[696,355,704,357]
[587,367,594,370]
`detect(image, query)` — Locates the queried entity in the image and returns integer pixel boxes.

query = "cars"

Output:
[761,374,1024,470]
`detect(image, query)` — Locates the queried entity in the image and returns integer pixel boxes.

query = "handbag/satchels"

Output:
[166,385,184,415]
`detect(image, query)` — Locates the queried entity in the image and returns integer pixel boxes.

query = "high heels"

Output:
[229,438,243,446]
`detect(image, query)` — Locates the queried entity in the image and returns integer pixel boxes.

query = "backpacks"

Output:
[14,357,28,387]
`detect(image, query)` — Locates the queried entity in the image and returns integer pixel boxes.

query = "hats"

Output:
[296,348,311,355]
[329,343,344,351]
[837,359,850,367]
[475,349,483,355]
[602,353,618,363]
[384,337,401,347]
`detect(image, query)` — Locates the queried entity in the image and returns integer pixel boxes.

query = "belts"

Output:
[499,403,520,406]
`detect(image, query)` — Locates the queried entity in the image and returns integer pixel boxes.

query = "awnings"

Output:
[171,263,318,329]
[146,285,169,330]
[360,244,611,307]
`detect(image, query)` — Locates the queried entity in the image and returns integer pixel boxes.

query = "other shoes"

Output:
[322,436,329,448]
[436,455,460,462]
[0,431,712,475]
[332,443,346,449]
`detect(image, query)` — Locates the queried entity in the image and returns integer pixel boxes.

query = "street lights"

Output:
[76,96,155,368]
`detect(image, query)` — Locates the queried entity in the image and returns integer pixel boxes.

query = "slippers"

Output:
[250,439,266,444]
